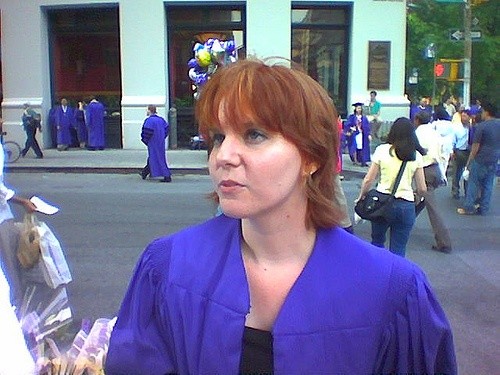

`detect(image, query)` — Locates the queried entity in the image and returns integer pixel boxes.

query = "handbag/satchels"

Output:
[355,189,398,223]
[14,212,73,289]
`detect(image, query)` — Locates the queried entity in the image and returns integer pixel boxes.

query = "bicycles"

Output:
[0,129,22,164]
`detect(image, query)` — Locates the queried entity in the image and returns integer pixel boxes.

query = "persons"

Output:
[102,54,458,375]
[136,105,170,183]
[336,111,344,180]
[354,117,427,259]
[20,104,43,159]
[49,96,104,151]
[365,91,382,139]
[346,103,371,167]
[0,117,44,309]
[406,91,500,215]
[412,110,453,254]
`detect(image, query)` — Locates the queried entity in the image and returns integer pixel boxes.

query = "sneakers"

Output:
[457,208,480,215]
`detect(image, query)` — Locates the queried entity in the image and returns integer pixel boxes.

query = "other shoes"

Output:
[433,244,452,252]
[160,177,171,182]
[340,176,344,180]
[138,170,146,180]
[353,162,368,167]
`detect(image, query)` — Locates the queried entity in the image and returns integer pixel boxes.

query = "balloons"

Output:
[188,39,237,100]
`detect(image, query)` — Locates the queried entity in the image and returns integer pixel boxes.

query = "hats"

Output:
[352,102,364,107]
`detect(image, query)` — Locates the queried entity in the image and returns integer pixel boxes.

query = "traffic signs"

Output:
[449,28,482,43]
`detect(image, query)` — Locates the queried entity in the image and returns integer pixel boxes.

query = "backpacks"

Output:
[30,113,42,127]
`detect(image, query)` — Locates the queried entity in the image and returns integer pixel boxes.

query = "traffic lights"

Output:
[435,61,459,80]
